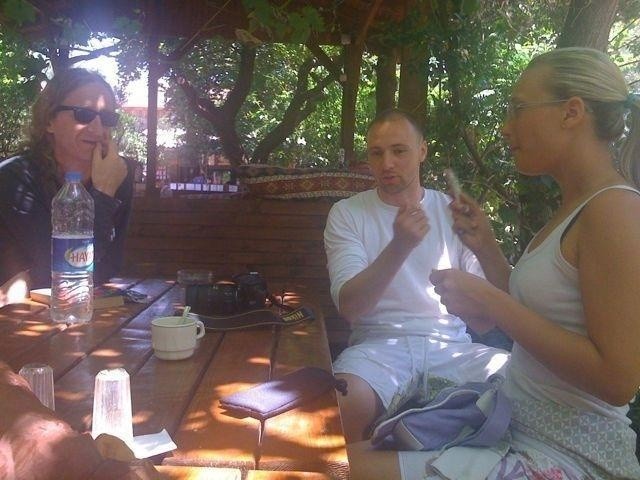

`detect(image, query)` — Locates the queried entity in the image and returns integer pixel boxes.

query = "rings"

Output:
[80,432,138,480]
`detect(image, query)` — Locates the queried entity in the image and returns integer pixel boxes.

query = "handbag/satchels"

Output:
[370,372,512,448]
[224,365,346,421]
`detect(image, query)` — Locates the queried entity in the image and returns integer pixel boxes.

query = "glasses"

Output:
[53,104,120,128]
[504,100,565,114]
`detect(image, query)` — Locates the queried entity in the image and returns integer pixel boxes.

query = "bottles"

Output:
[93,368,133,450]
[19,363,54,413]
[49,172,95,324]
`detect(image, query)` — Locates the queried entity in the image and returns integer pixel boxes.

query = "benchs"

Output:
[120,187,358,351]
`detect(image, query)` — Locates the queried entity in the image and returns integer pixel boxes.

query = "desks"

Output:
[1,273,352,480]
[117,462,333,480]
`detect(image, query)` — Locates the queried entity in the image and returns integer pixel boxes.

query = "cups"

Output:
[152,315,205,358]
[176,269,213,306]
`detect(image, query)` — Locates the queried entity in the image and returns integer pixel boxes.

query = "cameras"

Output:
[185,272,266,316]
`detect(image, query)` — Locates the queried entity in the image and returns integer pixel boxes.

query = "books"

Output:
[27,280,129,311]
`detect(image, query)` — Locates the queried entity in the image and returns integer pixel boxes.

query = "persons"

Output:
[346,47,639,480]
[322,109,518,439]
[0,361,171,480]
[1,66,137,307]
